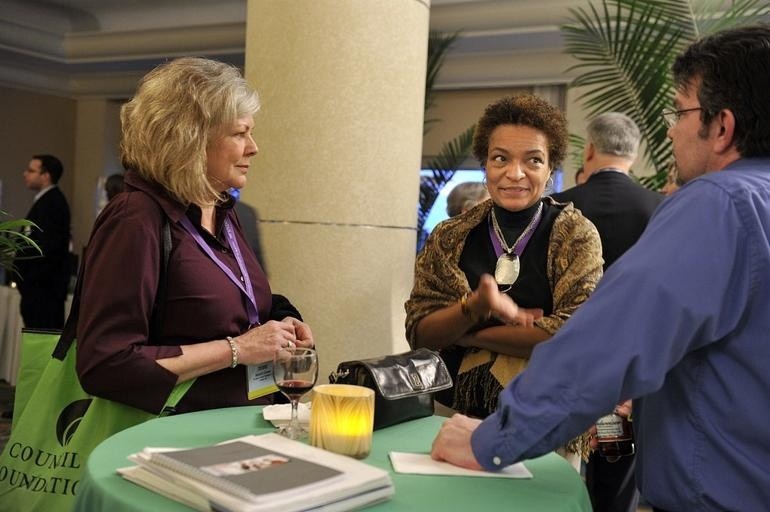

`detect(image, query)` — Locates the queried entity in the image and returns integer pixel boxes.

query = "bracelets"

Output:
[224,333,239,370]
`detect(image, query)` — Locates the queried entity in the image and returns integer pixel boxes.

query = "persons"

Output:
[77,58,318,415]
[9,154,71,332]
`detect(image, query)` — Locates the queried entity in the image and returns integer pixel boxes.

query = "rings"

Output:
[287,339,293,348]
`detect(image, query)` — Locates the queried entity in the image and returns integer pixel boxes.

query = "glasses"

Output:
[660,107,704,128]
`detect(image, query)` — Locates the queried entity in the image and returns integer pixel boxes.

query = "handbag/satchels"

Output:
[0,327,198,512]
[329,347,453,431]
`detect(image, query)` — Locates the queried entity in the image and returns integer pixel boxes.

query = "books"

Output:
[115,432,397,512]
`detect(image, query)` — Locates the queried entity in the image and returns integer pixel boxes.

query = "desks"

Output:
[87,399,588,512]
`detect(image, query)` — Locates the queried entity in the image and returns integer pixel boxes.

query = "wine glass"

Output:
[272,346,319,441]
